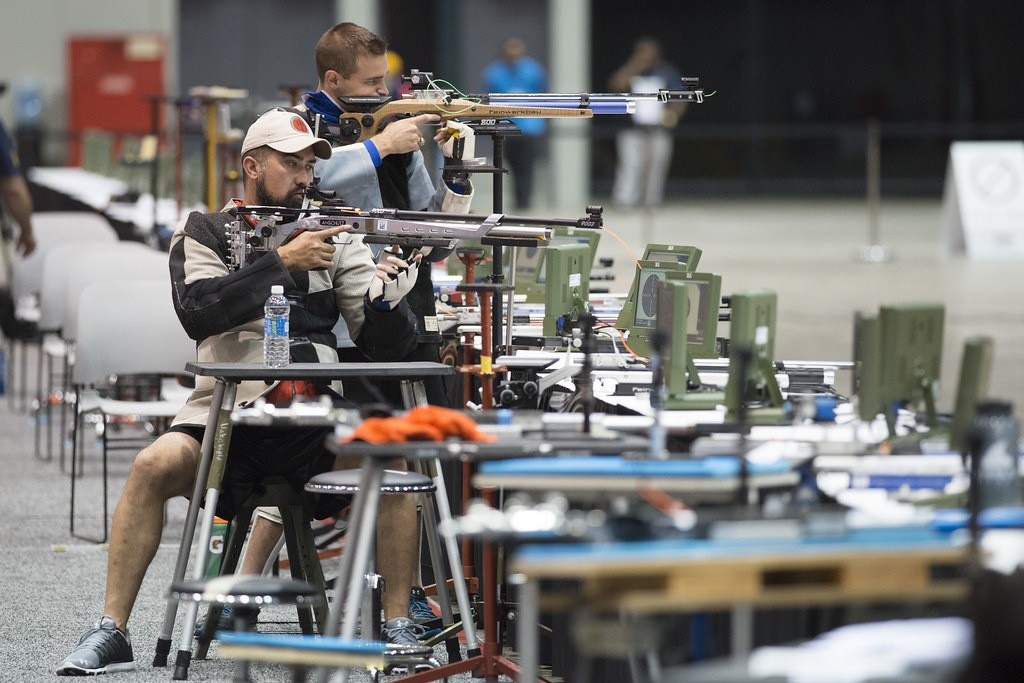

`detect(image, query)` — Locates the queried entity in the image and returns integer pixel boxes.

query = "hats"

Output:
[240,109,332,160]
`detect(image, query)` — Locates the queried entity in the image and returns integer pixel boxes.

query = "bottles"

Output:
[205,516,229,584]
[264,285,290,369]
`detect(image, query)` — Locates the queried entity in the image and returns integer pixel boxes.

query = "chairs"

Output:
[15,211,199,543]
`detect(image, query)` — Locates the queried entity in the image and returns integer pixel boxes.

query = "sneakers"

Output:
[382,616,441,674]
[408,586,437,625]
[56,616,136,675]
[193,603,258,639]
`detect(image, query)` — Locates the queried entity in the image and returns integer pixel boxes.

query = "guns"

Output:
[224,175,605,274]
[313,68,707,147]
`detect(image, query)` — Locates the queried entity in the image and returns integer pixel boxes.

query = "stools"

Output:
[192,475,315,660]
[305,469,449,683]
[168,575,324,683]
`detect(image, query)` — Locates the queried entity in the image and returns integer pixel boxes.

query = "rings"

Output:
[419,137,425,147]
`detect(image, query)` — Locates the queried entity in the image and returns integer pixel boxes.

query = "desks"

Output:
[154,363,482,680]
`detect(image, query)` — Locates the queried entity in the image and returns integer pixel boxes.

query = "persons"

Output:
[0,84,35,395]
[478,34,549,208]
[55,22,475,678]
[612,36,690,211]
